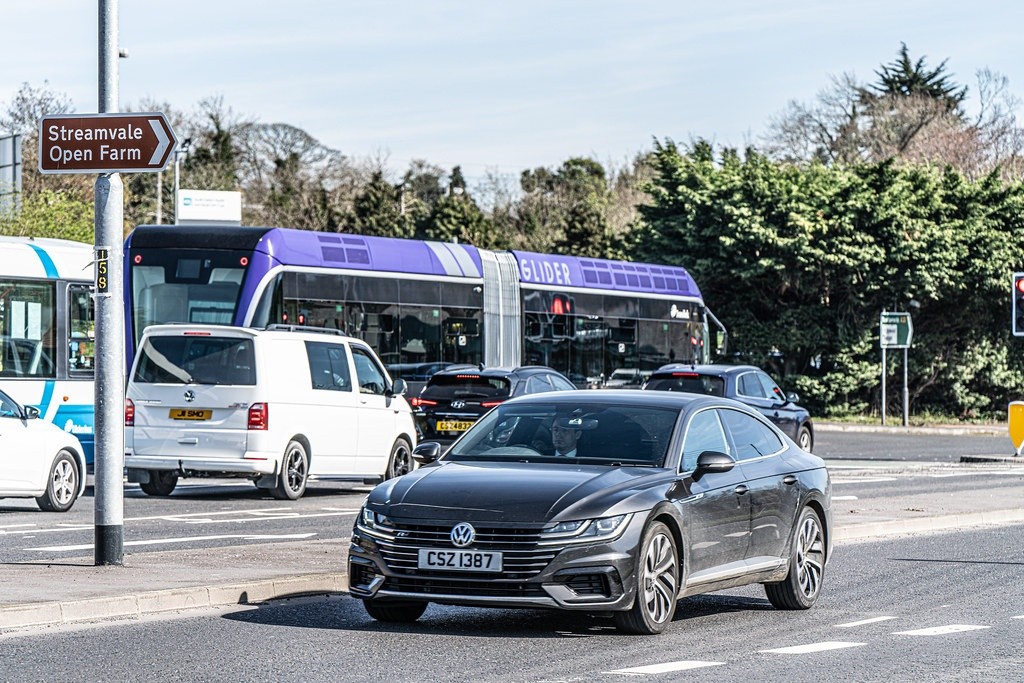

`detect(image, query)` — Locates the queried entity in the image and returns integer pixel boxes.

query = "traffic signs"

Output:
[880,311,914,348]
[38,111,179,175]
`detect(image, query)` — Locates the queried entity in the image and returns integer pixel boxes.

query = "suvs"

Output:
[640,362,815,455]
[410,363,583,455]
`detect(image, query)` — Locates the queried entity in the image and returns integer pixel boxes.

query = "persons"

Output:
[543,412,588,458]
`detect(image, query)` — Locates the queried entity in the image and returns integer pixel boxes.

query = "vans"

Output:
[126,323,420,500]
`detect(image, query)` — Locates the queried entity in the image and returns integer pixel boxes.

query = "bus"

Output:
[122,224,728,400]
[0,236,96,466]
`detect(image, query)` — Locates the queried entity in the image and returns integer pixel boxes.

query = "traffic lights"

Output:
[1012,271,1024,336]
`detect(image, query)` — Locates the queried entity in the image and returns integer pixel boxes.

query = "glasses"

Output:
[549,427,577,433]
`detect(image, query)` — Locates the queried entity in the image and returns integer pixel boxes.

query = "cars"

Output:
[0,338,54,378]
[605,367,654,388]
[347,387,832,636]
[0,390,86,512]
